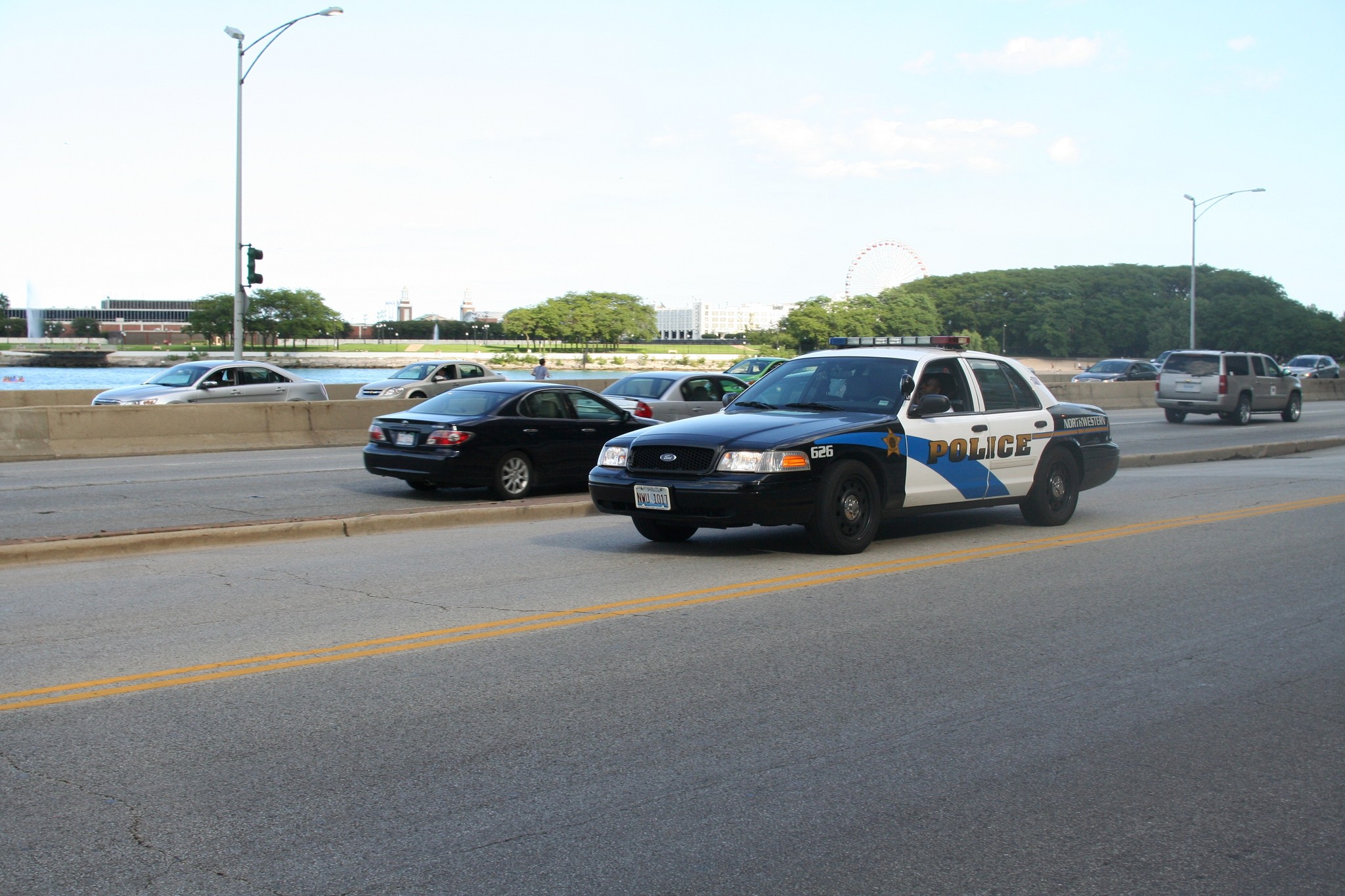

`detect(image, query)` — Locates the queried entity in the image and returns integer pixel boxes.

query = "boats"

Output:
[3,375,24,383]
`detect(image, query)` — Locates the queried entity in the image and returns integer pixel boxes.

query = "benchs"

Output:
[245,371,277,384]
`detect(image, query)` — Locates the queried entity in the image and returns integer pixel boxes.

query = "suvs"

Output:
[1153,348,1302,422]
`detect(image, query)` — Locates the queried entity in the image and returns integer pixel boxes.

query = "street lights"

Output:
[376,323,386,344]
[318,329,322,346]
[226,6,344,364]
[1184,187,1265,351]
[4,323,10,343]
[62,328,65,344]
[472,325,478,345]
[333,318,337,347]
[326,332,329,353]
[395,333,400,351]
[482,325,490,345]
[120,327,123,351]
[465,332,469,352]
[388,327,393,345]
[87,325,91,344]
[362,323,367,345]
[45,324,56,345]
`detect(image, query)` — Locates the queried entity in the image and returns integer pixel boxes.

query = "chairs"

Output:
[226,370,234,386]
[935,372,965,412]
[693,386,716,401]
[467,369,478,378]
[536,401,563,418]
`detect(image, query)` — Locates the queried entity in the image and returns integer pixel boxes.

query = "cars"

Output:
[88,359,328,405]
[356,361,508,401]
[1072,358,1162,382]
[705,355,794,401]
[590,332,1119,551]
[1282,354,1339,378]
[577,370,765,422]
[363,380,666,497]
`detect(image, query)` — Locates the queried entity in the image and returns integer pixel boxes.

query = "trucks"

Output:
[192,336,223,346]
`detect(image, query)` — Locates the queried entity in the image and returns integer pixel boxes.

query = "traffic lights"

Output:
[246,246,264,286]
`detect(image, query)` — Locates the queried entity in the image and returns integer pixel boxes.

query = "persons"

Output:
[896,375,954,415]
[1077,363,1081,370]
[436,367,449,380]
[531,358,550,380]
